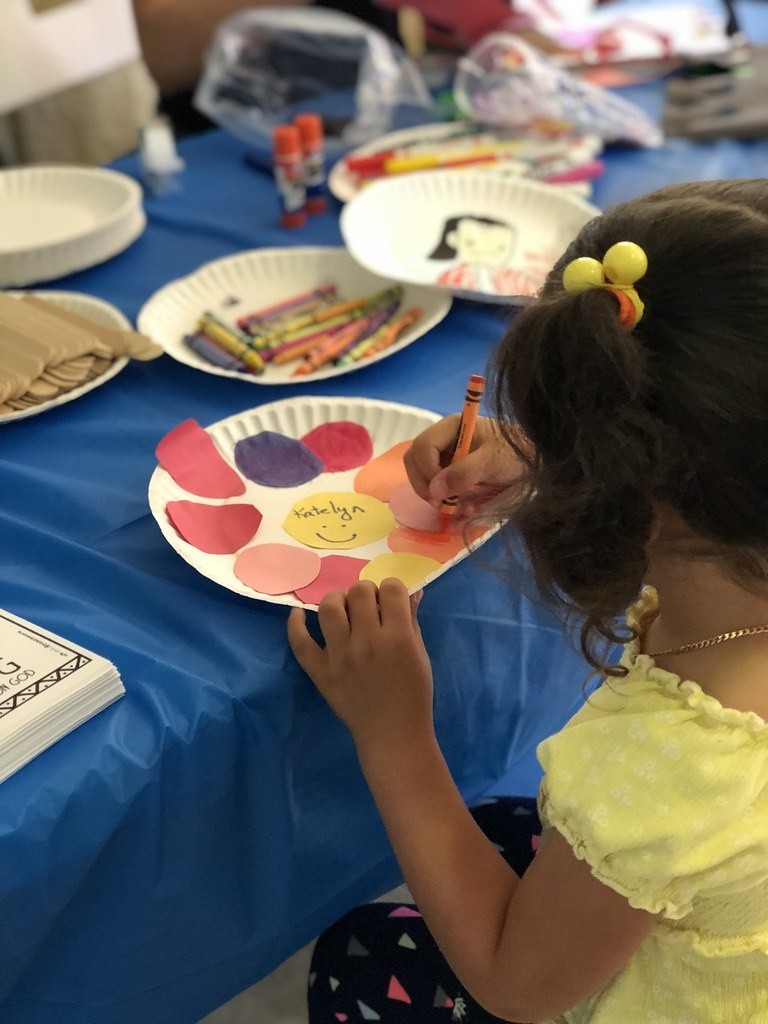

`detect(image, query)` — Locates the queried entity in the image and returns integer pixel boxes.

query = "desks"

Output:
[0,0,768,1024]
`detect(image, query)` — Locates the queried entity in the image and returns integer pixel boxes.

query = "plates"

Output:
[328,122,594,206]
[0,288,135,420]
[136,247,453,384]
[148,394,537,613]
[338,168,605,305]
[0,165,147,286]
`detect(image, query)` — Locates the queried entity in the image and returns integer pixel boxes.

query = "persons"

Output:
[660,44,768,143]
[286,174,768,1024]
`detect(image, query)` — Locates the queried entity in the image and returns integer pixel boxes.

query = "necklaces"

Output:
[640,608,767,657]
